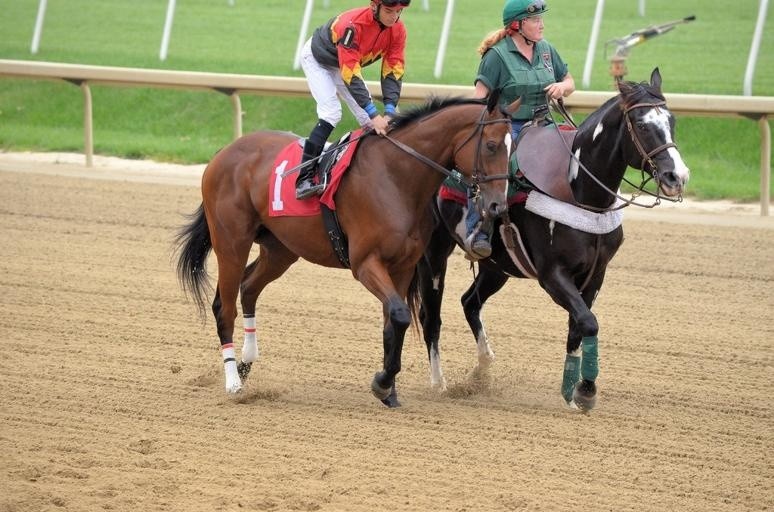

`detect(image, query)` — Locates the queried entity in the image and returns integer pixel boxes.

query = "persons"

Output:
[461,0,577,262]
[291,1,413,201]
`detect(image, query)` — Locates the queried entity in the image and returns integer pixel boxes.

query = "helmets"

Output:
[368,0,409,10]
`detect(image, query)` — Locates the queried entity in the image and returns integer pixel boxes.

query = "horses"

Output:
[170,87,522,409]
[405,66,690,416]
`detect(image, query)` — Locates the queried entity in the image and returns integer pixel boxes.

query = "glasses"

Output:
[525,1,545,12]
[377,0,409,7]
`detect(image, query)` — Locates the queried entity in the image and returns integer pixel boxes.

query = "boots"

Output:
[296,140,328,199]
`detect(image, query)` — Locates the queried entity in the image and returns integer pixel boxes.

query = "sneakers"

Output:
[465,239,491,263]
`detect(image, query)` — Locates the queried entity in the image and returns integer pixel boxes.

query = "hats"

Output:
[502,2,549,25]
